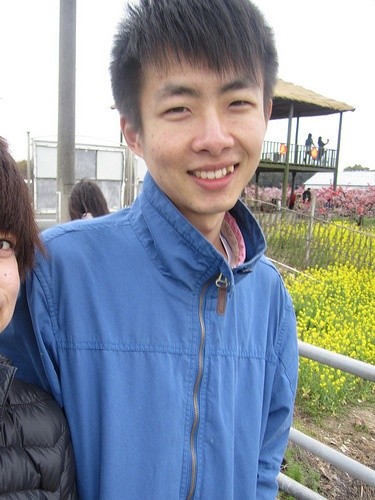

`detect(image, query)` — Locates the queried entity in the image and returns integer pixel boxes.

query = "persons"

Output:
[68,178,109,221]
[289,188,312,209]
[0,1,298,500]
[0,137,78,500]
[303,132,330,162]
